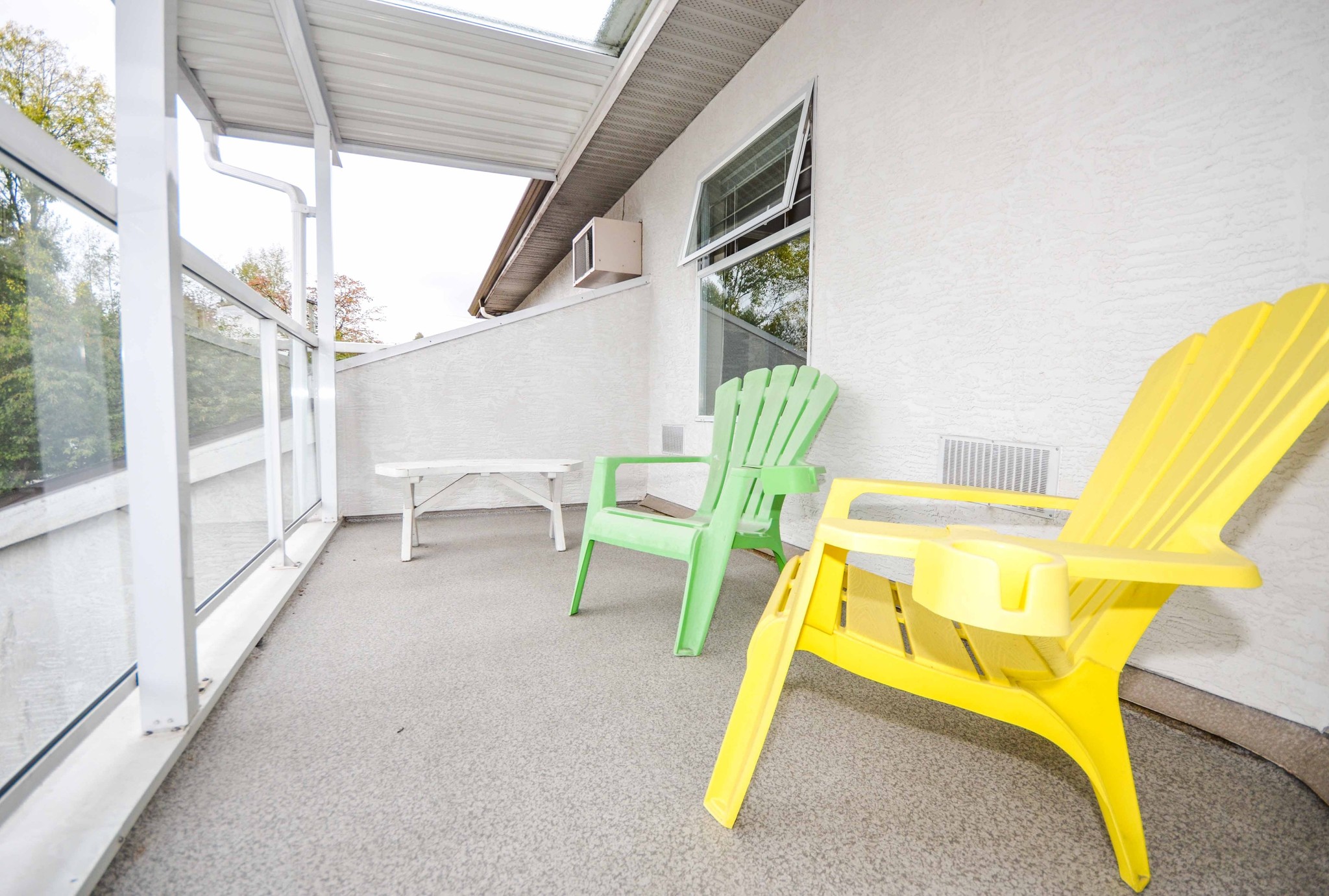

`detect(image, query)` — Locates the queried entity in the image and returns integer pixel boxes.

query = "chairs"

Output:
[699,281,1329,894]
[568,364,838,656]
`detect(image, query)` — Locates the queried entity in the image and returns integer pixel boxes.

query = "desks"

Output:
[373,459,585,563]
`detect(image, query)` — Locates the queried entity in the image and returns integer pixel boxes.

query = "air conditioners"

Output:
[572,216,643,289]
